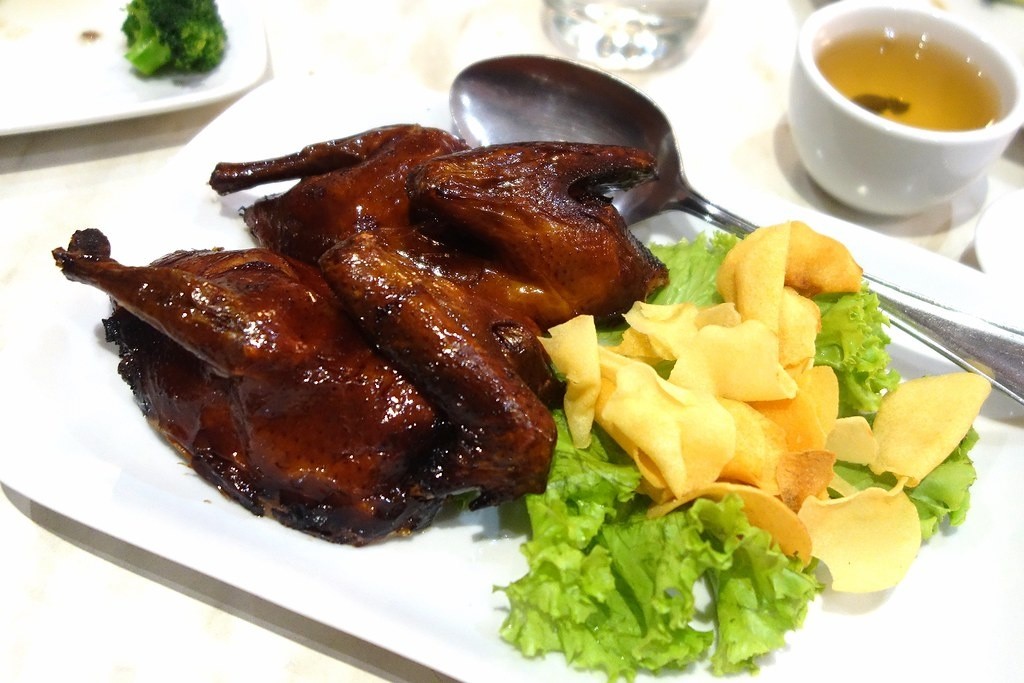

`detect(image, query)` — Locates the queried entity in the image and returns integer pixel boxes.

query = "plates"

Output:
[0,65,1024,683]
[0,0,269,137]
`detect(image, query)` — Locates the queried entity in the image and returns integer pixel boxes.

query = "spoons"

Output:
[447,54,1024,409]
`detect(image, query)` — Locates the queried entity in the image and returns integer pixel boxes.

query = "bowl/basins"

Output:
[786,0,1024,216]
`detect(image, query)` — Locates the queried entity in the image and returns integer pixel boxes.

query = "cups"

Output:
[538,0,708,75]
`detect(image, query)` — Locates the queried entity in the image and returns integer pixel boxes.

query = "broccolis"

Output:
[122,0,231,75]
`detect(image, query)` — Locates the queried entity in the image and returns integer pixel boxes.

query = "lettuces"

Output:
[490,233,979,683]
